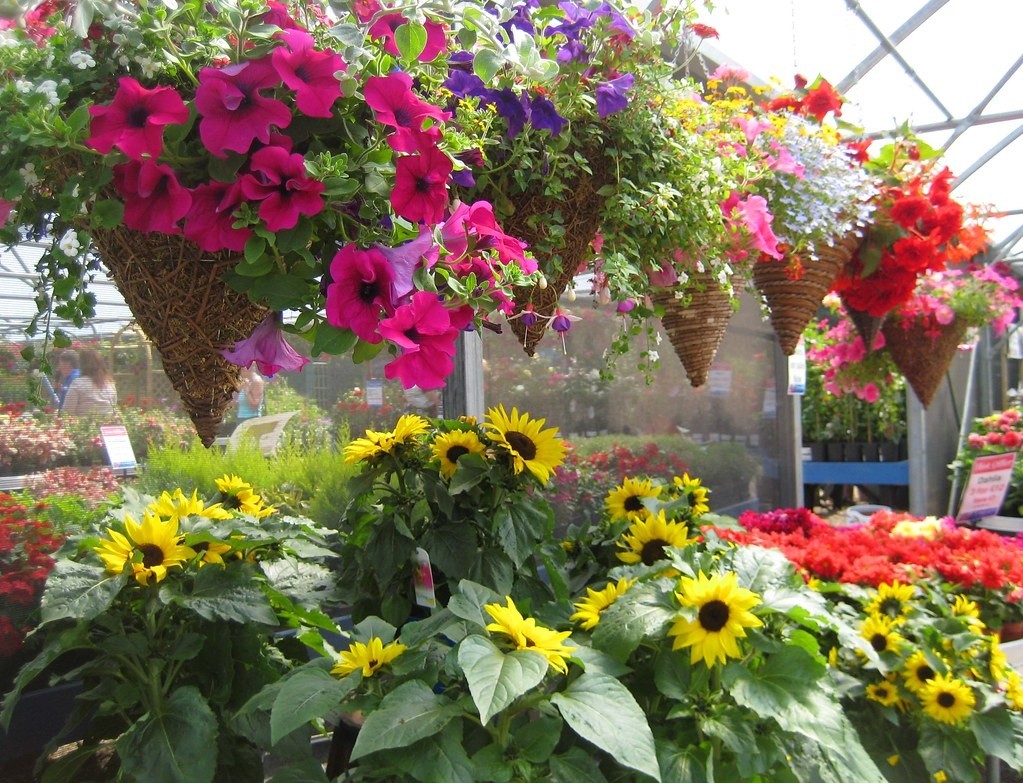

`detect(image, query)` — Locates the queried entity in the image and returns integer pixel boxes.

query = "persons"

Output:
[54,349,84,421]
[60,348,118,420]
[235,362,265,428]
[403,383,441,422]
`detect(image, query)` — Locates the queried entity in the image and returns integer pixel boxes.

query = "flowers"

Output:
[798,318,910,442]
[1,0,1022,387]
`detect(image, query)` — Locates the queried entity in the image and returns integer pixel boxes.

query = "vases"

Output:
[882,314,969,411]
[807,439,910,463]
[47,142,286,451]
